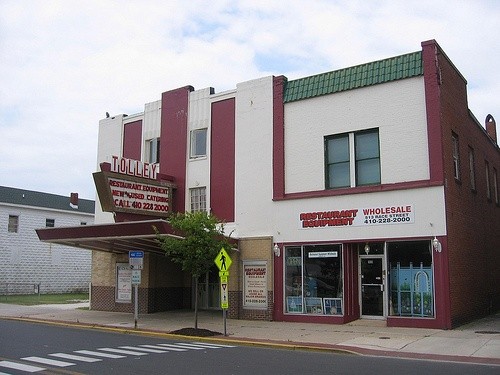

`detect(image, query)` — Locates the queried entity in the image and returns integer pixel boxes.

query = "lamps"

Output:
[433,236,442,252]
[273,242,280,257]
[364,241,370,256]
